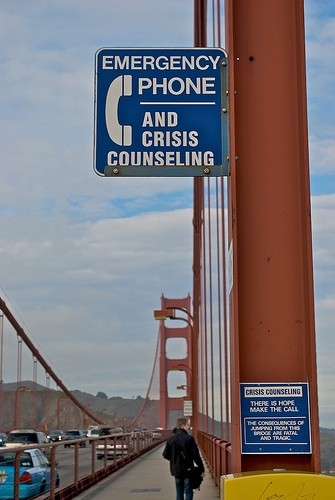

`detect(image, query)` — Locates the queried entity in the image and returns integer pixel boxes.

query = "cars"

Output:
[0,433,7,446]
[0,446,60,500]
[87,427,163,461]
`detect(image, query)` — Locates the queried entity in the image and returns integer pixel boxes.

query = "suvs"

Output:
[48,430,66,443]
[64,430,87,448]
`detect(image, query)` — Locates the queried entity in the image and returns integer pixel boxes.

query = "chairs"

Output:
[20,456,31,467]
[4,456,13,466]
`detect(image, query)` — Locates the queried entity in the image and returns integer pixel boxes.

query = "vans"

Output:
[3,429,47,447]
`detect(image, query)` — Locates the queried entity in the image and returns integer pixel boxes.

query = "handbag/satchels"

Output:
[188,466,203,489]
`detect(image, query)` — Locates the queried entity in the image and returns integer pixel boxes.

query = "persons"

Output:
[163,418,205,500]
